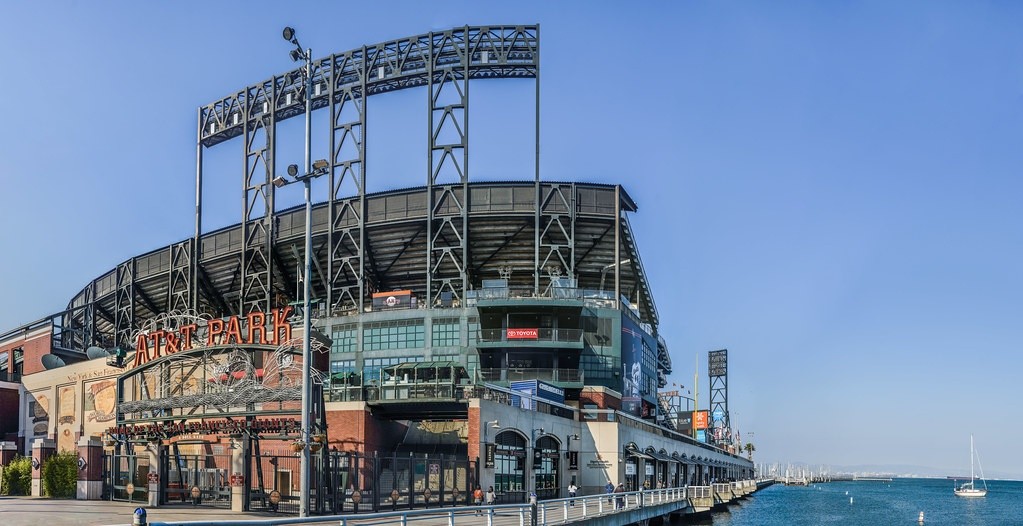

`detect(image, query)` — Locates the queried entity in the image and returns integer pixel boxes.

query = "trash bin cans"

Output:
[363,385,379,401]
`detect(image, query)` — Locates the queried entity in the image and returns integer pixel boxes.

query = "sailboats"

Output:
[954,434,987,498]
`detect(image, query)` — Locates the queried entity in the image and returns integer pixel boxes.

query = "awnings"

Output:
[751,468,759,472]
[626,448,732,469]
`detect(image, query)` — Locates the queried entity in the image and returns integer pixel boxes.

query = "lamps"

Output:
[487,420,501,436]
[532,427,546,449]
[566,434,580,450]
[614,371,619,376]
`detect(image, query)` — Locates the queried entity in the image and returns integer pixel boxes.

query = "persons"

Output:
[568,481,581,509]
[711,477,750,483]
[643,480,672,495]
[486,486,497,516]
[474,485,484,517]
[614,483,624,511]
[605,481,615,506]
